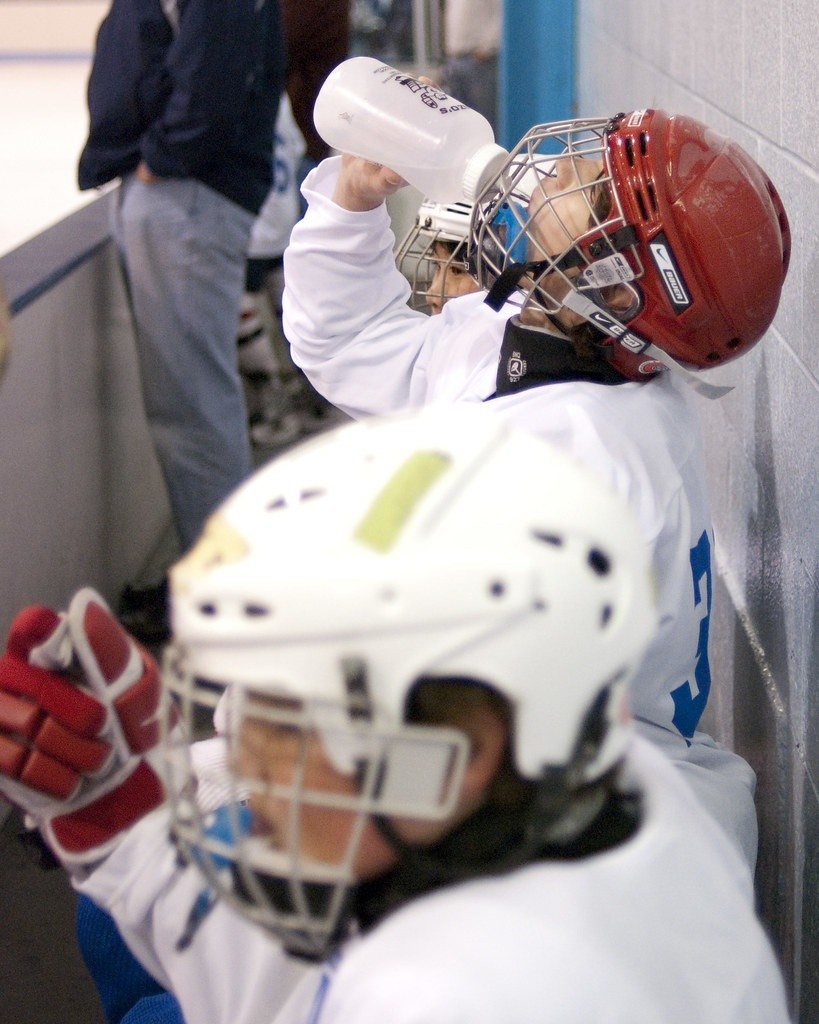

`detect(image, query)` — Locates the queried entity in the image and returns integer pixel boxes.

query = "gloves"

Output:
[0,586,184,877]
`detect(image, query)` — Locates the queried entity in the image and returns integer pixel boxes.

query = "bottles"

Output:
[313,57,515,209]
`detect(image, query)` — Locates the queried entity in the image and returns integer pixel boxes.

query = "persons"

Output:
[0,406,791,1024]
[281,73,793,917]
[77,0,353,650]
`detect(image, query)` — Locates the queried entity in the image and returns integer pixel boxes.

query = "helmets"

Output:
[465,108,791,397]
[392,148,558,317]
[156,397,657,962]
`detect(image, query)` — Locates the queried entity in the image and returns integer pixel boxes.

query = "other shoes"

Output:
[118,579,169,644]
[247,407,301,451]
